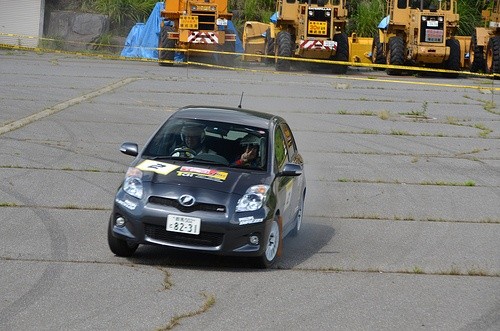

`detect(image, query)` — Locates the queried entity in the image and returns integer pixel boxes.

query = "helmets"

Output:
[181,125,206,144]
[240,134,262,156]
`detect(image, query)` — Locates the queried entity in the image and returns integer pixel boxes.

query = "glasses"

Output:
[244,144,258,150]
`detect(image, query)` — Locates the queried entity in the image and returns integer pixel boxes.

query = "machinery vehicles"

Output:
[158,0,236,67]
[241,0,350,75]
[350,0,462,76]
[457,0,500,77]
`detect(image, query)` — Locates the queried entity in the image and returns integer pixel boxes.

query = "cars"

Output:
[107,105,308,270]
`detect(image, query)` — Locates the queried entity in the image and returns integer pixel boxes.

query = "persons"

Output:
[171,125,215,158]
[230,135,263,167]
